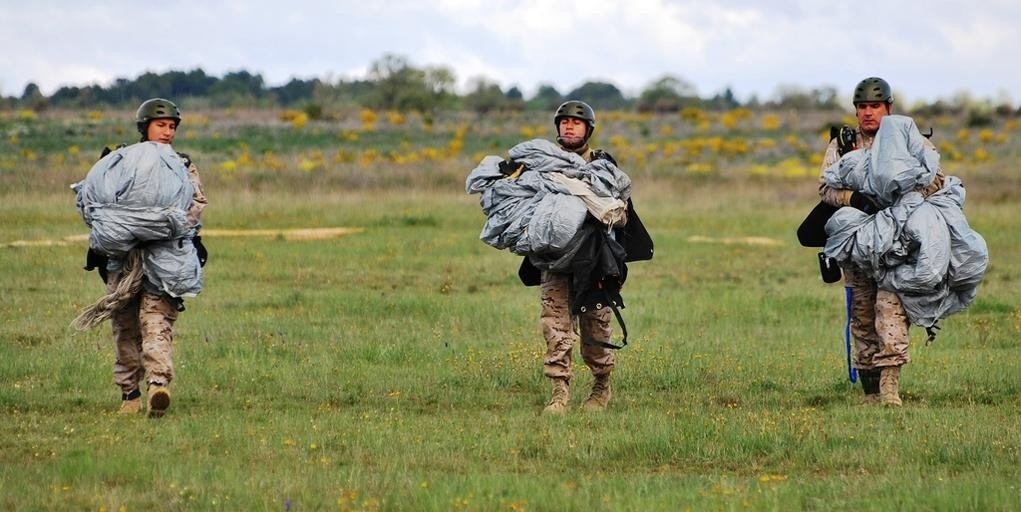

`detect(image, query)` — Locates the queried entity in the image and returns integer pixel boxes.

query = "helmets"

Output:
[135,99,181,125]
[553,101,596,129]
[852,77,892,105]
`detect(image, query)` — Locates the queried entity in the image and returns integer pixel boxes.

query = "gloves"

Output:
[850,189,877,216]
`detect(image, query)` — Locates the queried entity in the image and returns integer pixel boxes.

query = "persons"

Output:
[96,98,209,417]
[819,76,947,404]
[501,100,628,415]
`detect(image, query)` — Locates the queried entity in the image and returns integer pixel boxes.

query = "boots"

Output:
[582,373,611,411]
[543,375,568,413]
[862,367,902,407]
[146,382,170,418]
[116,384,143,414]
